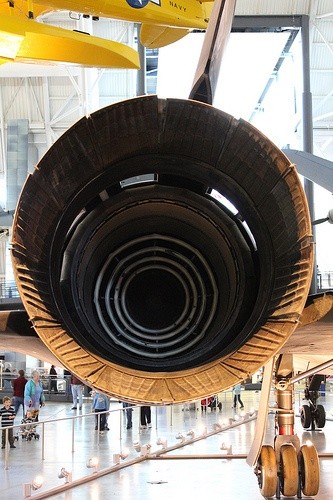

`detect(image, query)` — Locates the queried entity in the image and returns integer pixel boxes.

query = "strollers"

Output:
[13,405,39,442]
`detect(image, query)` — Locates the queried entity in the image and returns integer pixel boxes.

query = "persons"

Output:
[71,374,85,410]
[0,395,16,449]
[91,388,110,430]
[122,402,134,429]
[24,370,45,428]
[232,383,244,408]
[200,399,207,413]
[12,369,28,418]
[21,410,35,432]
[3,364,59,392]
[140,406,152,429]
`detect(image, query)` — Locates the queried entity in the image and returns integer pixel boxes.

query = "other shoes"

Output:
[140,425,147,429]
[127,425,132,429]
[79,407,81,410]
[72,407,78,409]
[1,446,5,449]
[148,423,152,428]
[10,445,18,449]
[105,426,109,430]
[95,426,98,430]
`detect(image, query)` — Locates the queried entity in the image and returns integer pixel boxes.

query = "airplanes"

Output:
[0,1,332,499]
